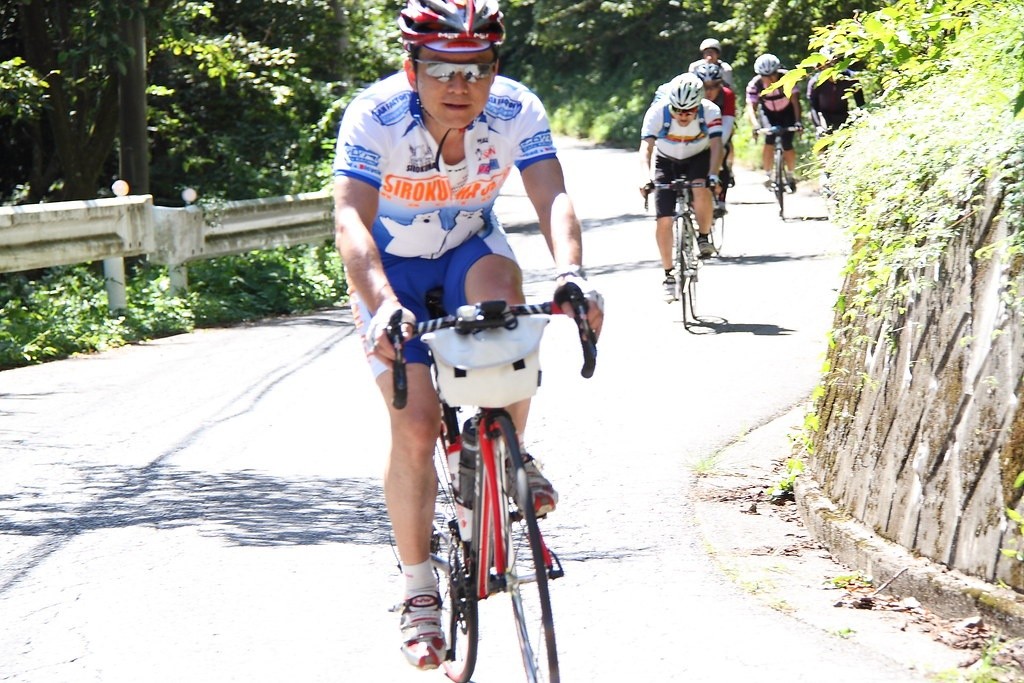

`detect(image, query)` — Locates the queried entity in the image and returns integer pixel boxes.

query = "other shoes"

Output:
[727,177,736,188]
[713,209,728,219]
[768,182,776,192]
[782,178,796,194]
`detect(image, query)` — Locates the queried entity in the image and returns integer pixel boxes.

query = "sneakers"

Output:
[397,565,447,671]
[698,238,712,255]
[505,453,559,519]
[663,276,676,302]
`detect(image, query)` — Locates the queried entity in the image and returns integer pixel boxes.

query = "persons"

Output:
[807,47,865,136]
[638,73,723,287]
[652,38,735,218]
[745,54,801,192]
[332,0,603,670]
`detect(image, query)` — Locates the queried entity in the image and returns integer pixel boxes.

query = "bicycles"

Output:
[749,123,807,220]
[643,143,733,334]
[382,278,598,683]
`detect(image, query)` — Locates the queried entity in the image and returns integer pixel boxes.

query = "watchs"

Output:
[555,265,588,280]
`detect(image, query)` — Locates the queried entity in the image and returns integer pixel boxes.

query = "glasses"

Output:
[674,111,696,116]
[414,58,496,85]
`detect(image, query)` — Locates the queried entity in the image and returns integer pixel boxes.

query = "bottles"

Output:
[447,435,475,542]
[460,417,479,506]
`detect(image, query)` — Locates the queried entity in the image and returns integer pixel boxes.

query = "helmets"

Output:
[819,46,846,61]
[695,63,724,82]
[397,0,506,53]
[753,54,780,75]
[669,73,704,109]
[699,38,721,52]
